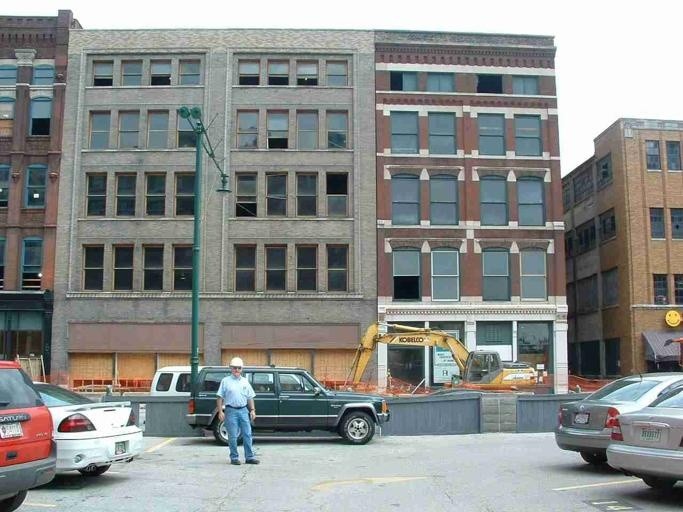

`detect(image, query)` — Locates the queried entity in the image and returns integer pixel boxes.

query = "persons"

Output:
[216,357,260,465]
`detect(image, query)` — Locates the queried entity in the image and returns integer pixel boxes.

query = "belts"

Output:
[225,405,247,409]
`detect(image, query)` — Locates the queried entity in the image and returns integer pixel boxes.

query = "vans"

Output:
[147,366,311,397]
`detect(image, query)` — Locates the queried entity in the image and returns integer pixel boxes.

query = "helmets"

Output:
[230,357,243,368]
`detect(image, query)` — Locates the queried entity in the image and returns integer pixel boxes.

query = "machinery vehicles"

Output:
[338,320,547,398]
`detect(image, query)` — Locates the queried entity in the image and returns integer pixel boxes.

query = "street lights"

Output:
[175,105,234,399]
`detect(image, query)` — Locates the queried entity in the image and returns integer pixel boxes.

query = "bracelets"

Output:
[250,409,255,410]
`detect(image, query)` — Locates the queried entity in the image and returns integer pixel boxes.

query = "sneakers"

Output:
[231,460,241,465]
[246,460,259,464]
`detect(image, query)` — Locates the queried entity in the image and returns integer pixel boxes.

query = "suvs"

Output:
[0,359,58,510]
[185,366,390,443]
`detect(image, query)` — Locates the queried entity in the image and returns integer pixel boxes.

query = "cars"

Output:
[556,369,682,473]
[25,376,148,496]
[603,382,682,491]
